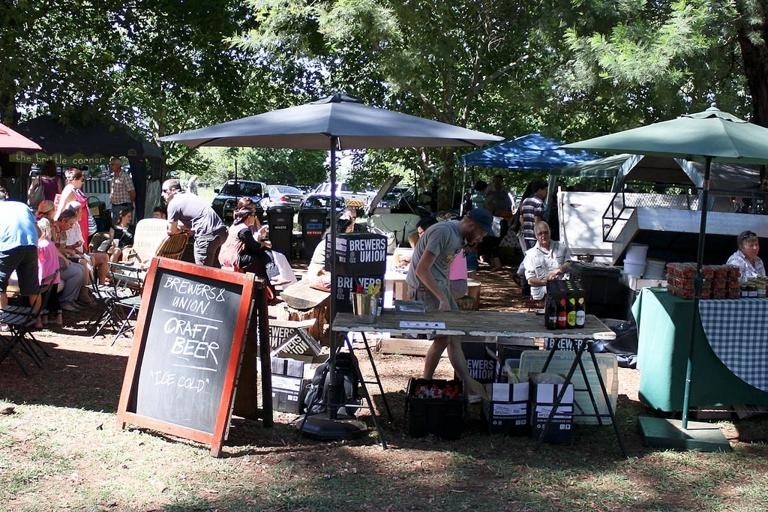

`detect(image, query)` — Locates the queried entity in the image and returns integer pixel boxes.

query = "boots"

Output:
[79,287,92,303]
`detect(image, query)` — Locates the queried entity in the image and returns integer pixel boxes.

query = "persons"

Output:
[409,172,576,311]
[0,153,277,322]
[725,230,766,283]
[401,205,494,392]
[303,216,356,295]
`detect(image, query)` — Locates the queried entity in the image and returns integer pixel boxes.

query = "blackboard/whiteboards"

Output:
[117,255,255,446]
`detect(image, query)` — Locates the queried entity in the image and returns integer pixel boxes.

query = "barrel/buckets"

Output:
[466,279,481,307]
[623,243,665,278]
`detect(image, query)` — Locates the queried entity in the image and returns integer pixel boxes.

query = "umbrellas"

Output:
[460,132,605,172]
[551,100,768,428]
[155,90,506,418]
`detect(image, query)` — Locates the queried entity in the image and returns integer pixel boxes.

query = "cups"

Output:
[350,294,376,324]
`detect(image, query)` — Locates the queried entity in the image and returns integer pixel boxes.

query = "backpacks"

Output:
[300,353,361,417]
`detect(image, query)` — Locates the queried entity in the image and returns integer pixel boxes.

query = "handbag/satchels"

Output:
[240,248,271,275]
[500,230,521,249]
[589,319,637,369]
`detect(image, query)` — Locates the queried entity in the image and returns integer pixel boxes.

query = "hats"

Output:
[469,209,493,232]
[38,199,54,213]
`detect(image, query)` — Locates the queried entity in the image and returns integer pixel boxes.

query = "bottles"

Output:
[353,274,384,318]
[546,277,585,329]
[82,179,111,193]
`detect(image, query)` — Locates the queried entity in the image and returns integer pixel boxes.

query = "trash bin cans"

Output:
[298,208,328,262]
[266,205,295,263]
[570,260,620,318]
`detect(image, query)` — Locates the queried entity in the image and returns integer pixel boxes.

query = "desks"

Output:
[297,311,629,458]
[638,285,768,414]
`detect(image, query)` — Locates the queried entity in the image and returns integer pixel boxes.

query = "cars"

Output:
[208,174,383,232]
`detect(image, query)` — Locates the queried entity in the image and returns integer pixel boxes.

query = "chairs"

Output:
[0,217,189,377]
